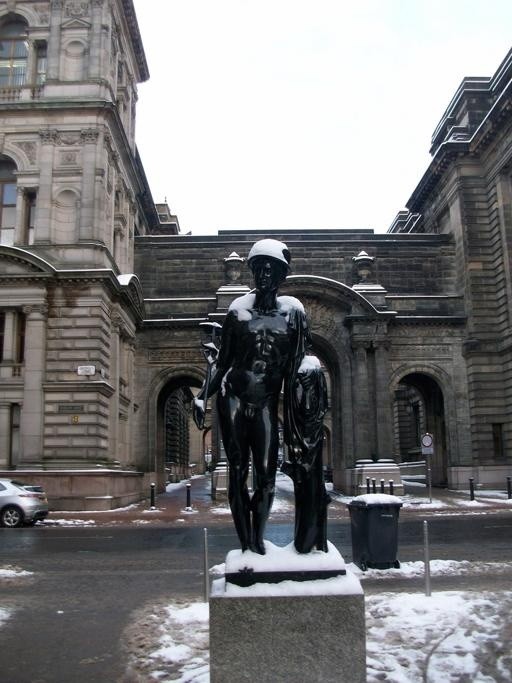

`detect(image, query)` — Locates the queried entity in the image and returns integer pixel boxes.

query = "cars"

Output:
[1,476,52,529]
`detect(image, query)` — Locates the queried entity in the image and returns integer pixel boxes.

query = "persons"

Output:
[192,237,312,554]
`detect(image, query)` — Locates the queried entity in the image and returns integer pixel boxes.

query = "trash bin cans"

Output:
[346,493,403,571]
